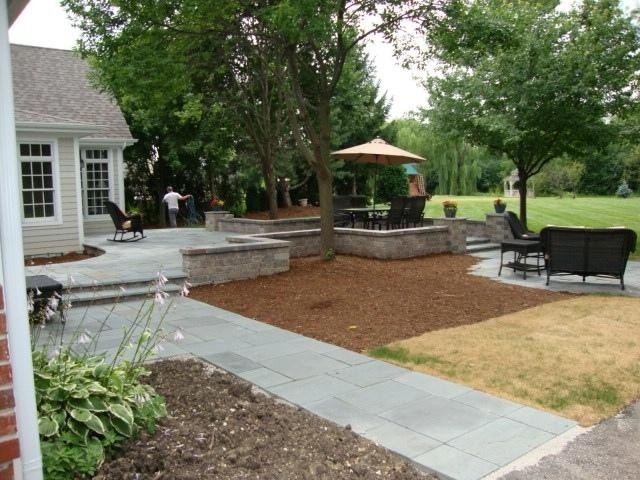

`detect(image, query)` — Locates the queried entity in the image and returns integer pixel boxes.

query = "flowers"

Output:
[28,265,192,480]
[443,201,457,208]
[495,199,501,205]
[210,195,225,208]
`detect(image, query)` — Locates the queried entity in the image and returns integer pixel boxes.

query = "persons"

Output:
[162,186,190,227]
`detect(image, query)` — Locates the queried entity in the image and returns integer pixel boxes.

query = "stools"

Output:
[26,275,65,322]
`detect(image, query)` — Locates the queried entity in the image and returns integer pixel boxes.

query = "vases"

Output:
[443,206,457,218]
[213,205,222,211]
[494,204,506,213]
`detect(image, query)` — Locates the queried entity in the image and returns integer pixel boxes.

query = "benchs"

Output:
[538,226,637,290]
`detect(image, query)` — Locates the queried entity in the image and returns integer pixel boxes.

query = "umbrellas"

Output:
[330,136,427,216]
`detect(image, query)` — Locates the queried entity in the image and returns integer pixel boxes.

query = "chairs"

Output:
[333,194,427,231]
[504,211,542,272]
[103,201,147,242]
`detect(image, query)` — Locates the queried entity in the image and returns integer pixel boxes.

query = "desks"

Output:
[498,239,541,280]
